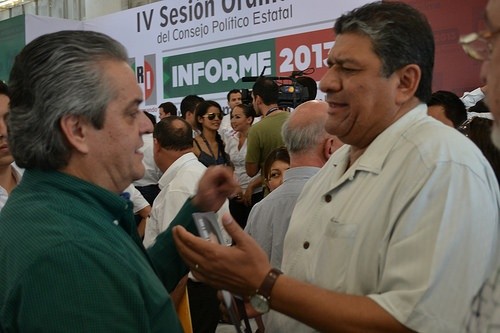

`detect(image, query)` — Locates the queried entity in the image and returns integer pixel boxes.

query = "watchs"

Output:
[249,268,283,316]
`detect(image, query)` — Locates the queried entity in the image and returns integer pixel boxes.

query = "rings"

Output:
[193,263,199,270]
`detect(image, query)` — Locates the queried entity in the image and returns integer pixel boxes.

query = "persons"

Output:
[0,0,500,333]
[0,30,244,333]
[169,3,500,333]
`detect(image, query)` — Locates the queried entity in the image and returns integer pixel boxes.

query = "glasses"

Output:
[459,26,500,61]
[202,112,224,121]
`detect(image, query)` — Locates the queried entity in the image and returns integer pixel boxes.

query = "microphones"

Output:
[242,76,292,82]
